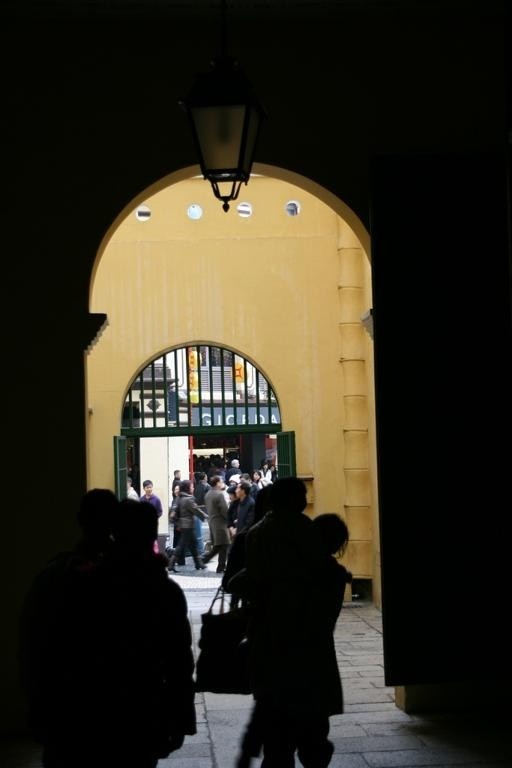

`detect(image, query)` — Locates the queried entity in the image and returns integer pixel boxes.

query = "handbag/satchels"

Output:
[195,589,269,694]
[222,559,266,597]
[168,503,180,523]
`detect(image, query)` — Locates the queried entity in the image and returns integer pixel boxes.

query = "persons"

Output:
[124,449,279,577]
[220,479,353,767]
[19,488,122,744]
[42,498,197,768]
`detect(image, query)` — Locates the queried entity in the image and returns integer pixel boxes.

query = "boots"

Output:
[193,555,207,570]
[168,554,179,573]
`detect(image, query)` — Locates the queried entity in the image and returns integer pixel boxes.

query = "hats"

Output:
[229,474,241,483]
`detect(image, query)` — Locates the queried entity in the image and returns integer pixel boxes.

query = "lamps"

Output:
[177,0,264,210]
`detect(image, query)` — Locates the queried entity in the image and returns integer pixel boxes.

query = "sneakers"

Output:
[217,567,226,573]
